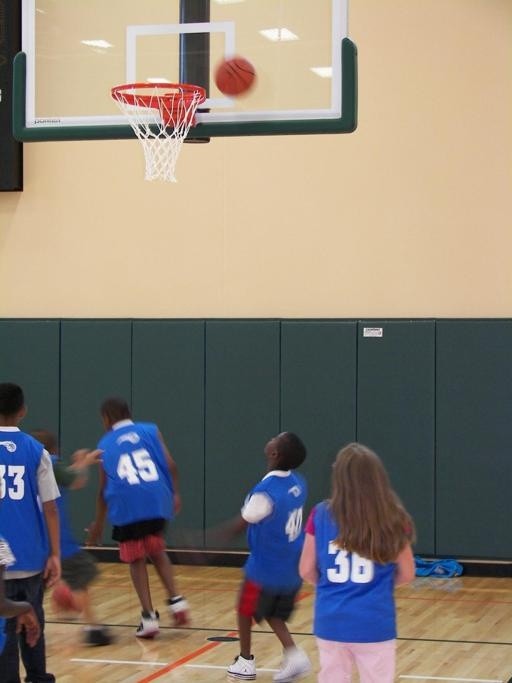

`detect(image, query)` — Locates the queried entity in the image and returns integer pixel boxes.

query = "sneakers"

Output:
[135,612,160,638]
[85,626,112,646]
[167,595,190,626]
[226,653,256,680]
[273,650,312,682]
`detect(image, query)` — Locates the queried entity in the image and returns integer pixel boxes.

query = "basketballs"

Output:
[216,57,255,97]
[51,582,83,620]
